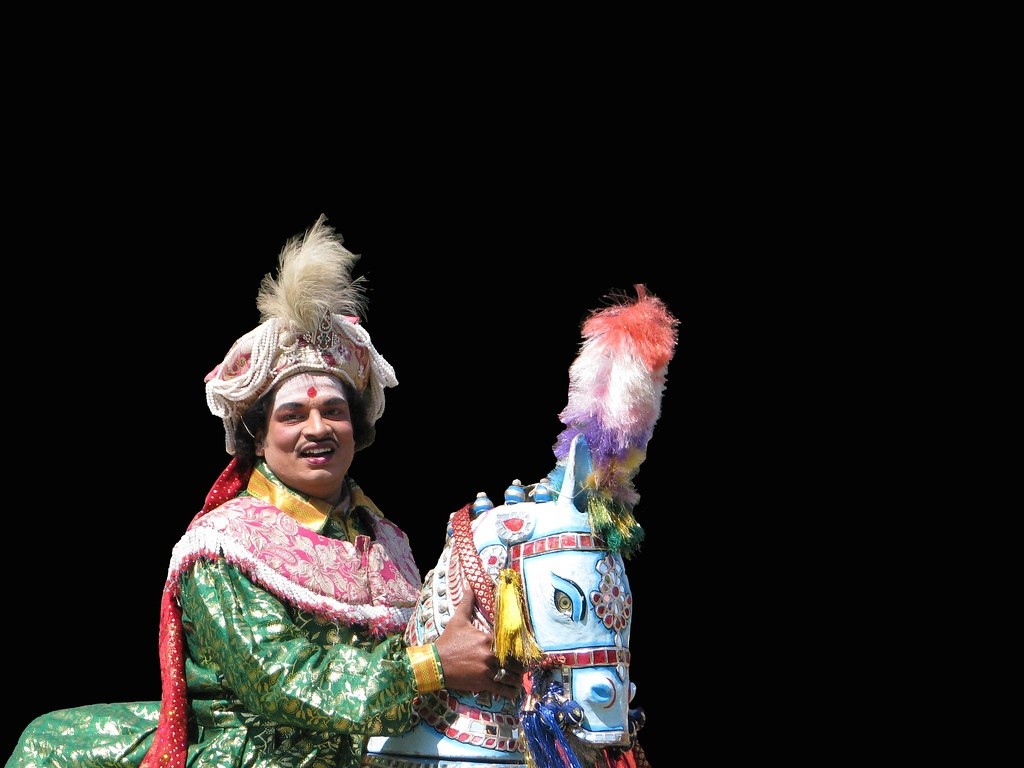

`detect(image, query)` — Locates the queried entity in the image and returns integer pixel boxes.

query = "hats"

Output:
[205,213,397,455]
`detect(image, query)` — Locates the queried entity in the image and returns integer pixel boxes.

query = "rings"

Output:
[492,669,506,680]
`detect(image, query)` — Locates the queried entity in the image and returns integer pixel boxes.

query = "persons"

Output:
[182,214,526,768]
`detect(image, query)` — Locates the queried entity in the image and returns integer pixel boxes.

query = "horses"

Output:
[363,434,640,768]
